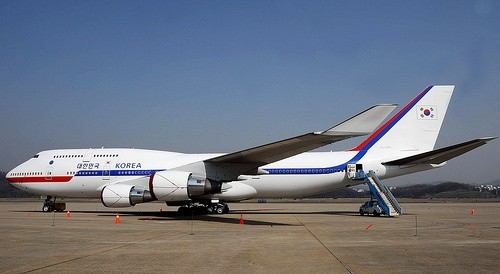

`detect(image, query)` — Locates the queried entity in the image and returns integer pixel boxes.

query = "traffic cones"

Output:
[470,207,475,216]
[159,209,163,216]
[239,214,245,225]
[115,213,120,224]
[65,209,71,218]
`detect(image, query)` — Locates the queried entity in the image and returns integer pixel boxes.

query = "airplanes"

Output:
[4,84,497,213]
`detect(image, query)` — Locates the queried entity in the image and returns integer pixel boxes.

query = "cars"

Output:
[359,200,384,216]
